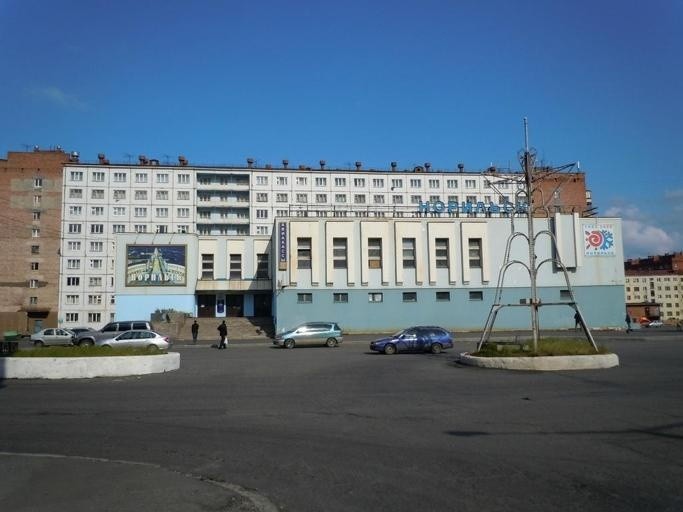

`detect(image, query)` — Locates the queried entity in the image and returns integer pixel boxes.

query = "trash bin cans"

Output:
[0,341,18,353]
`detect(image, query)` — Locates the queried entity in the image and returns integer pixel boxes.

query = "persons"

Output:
[190,320,199,342]
[625,311,632,333]
[216,319,227,349]
[573,310,581,332]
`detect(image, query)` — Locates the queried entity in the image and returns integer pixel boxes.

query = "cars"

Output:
[274,322,343,349]
[29,320,170,352]
[369,326,453,355]
[649,320,663,328]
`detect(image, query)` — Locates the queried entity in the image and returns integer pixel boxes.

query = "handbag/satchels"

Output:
[224,336,229,344]
